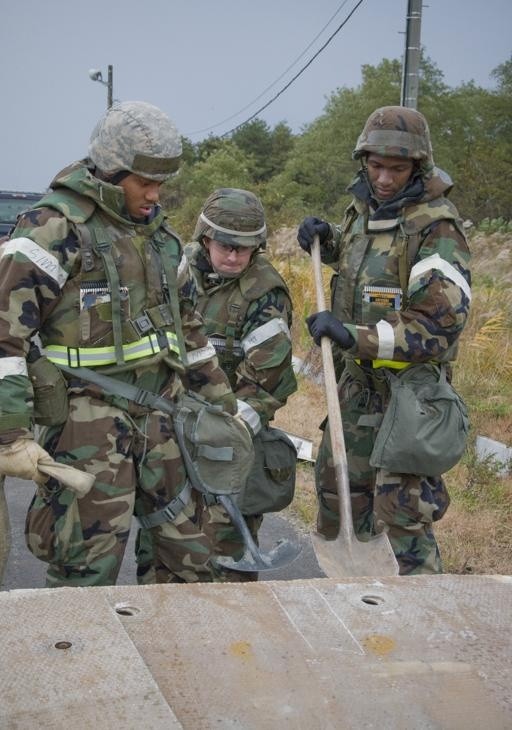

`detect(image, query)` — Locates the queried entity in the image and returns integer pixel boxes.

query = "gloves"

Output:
[297,217,331,252]
[305,311,356,351]
[1,436,56,486]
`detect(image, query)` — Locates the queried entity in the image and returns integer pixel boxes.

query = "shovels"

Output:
[307,235,400,578]
[216,494,302,572]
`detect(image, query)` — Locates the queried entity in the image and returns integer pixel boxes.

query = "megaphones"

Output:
[88,69,101,81]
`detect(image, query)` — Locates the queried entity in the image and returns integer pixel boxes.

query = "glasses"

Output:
[217,242,256,256]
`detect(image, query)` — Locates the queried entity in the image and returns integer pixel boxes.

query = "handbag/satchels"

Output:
[241,423,299,519]
[366,363,475,475]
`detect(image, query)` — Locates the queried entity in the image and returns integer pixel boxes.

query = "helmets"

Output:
[86,100,186,183]
[193,188,267,280]
[350,105,434,203]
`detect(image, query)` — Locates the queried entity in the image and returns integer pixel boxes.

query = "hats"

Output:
[53,361,252,497]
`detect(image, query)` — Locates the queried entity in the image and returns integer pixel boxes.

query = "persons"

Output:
[1,100,256,589]
[297,106,470,575]
[182,187,296,582]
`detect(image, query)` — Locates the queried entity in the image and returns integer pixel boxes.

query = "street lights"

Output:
[89,64,113,110]
